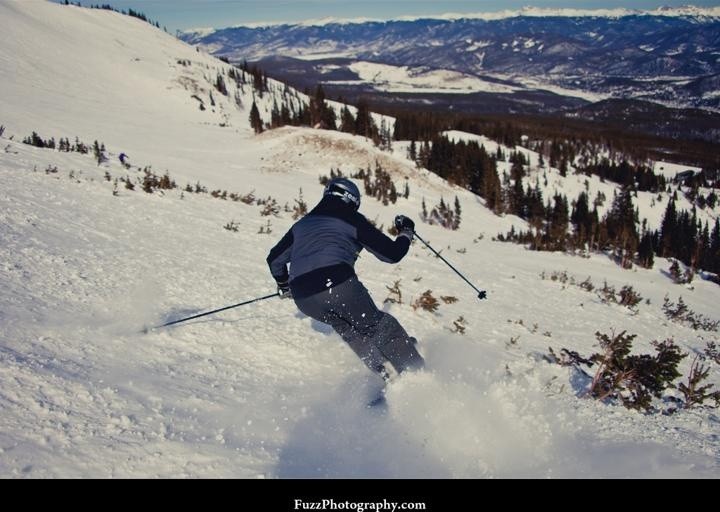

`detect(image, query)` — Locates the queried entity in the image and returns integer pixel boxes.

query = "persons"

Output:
[268,177,425,383]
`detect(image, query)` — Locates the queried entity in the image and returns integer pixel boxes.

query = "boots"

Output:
[373,343,429,387]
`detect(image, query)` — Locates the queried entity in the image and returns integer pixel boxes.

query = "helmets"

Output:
[323,177,361,211]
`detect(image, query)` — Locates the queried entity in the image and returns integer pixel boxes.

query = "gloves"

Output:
[394,214,415,243]
[276,278,293,300]
